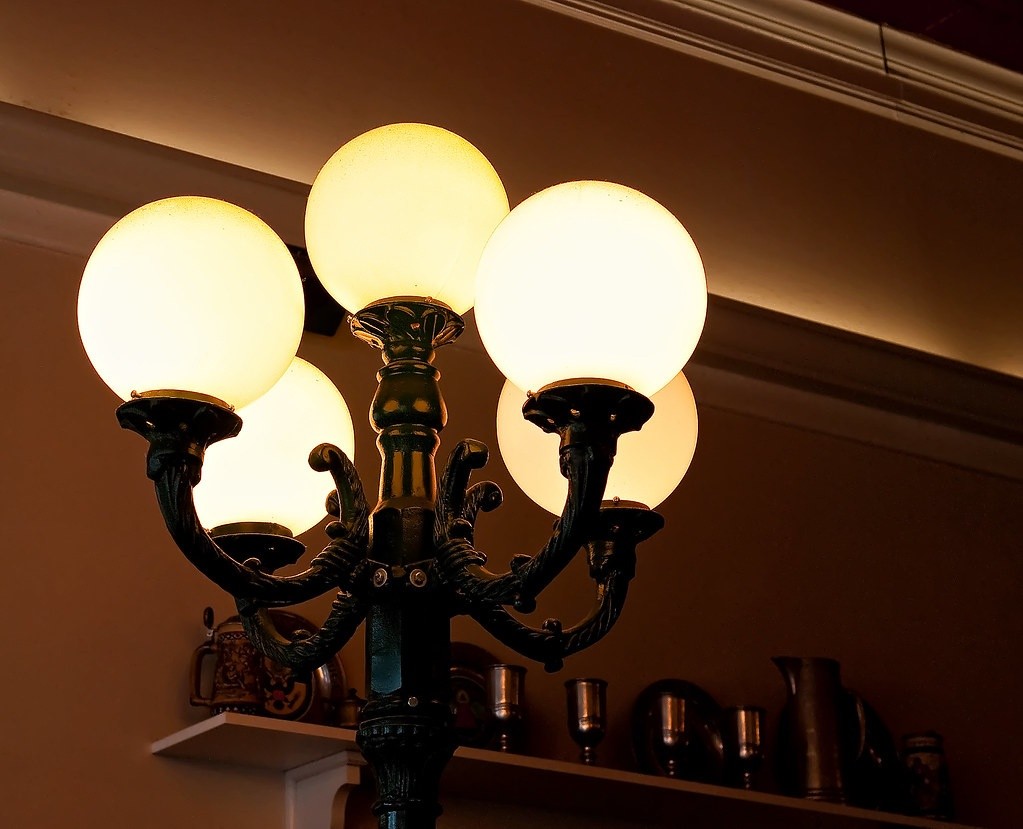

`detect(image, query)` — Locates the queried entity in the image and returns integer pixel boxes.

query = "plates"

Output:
[264,610,347,726]
[777,691,897,811]
[449,641,499,753]
[631,679,730,785]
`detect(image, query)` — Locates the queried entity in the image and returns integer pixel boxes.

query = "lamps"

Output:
[77,122,708,829]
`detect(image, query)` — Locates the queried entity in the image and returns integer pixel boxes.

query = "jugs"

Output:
[772,654,865,805]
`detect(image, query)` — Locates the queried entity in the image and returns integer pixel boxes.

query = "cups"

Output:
[191,617,263,717]
[905,731,951,823]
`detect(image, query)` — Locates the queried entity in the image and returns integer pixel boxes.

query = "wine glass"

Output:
[724,704,767,790]
[565,679,607,765]
[487,664,527,754]
[649,692,690,777]
